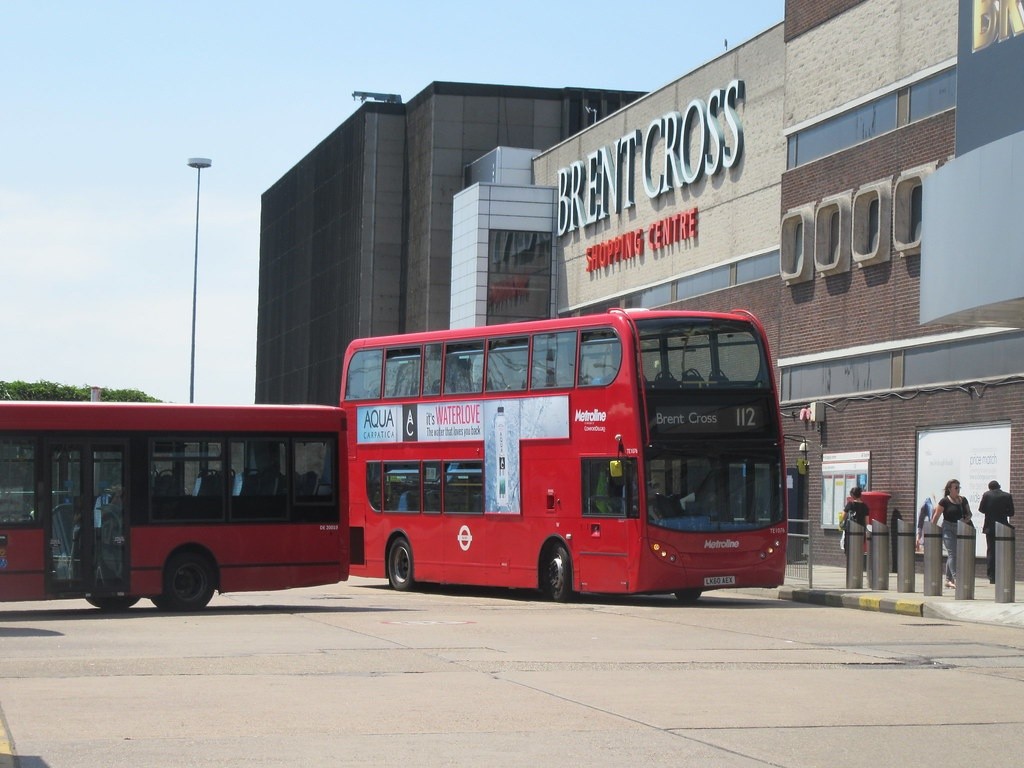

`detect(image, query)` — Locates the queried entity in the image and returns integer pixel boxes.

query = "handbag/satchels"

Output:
[961,517,974,528]
[840,510,853,529]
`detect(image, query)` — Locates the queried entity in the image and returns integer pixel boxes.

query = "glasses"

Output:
[948,486,961,490]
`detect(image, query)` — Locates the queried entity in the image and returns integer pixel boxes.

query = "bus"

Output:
[339,307,810,605]
[0,399,351,614]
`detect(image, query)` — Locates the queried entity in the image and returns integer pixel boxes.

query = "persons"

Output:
[931,479,972,589]
[840,487,870,538]
[622,464,656,517]
[979,480,1015,584]
[915,494,943,554]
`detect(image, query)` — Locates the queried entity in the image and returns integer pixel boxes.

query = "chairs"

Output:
[411,368,729,389]
[398,492,418,510]
[155,469,317,495]
